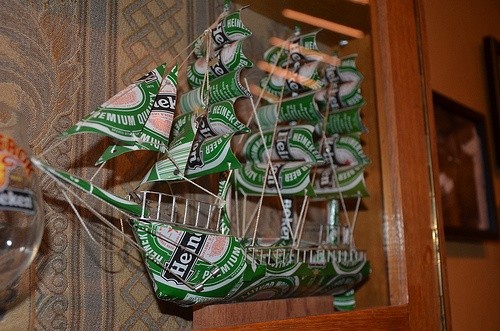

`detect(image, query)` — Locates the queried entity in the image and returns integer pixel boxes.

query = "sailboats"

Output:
[32,4,373,313]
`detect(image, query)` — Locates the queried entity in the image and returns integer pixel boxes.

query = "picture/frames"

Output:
[432,91,500,240]
[484,36,500,167]
